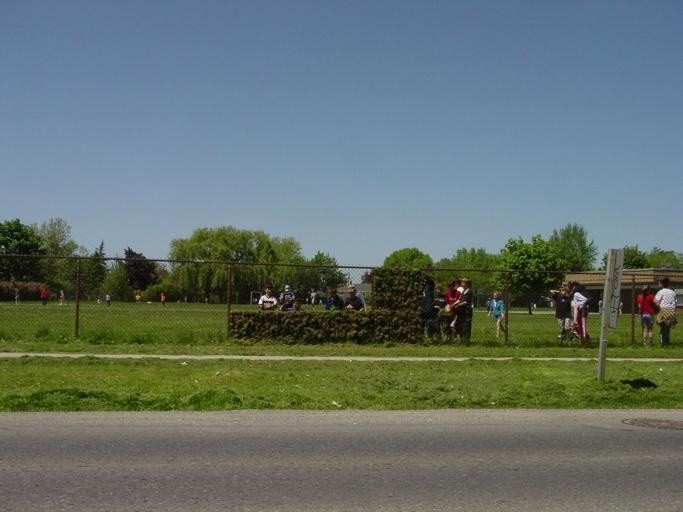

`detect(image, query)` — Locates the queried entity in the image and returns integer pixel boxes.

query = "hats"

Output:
[285,285,290,291]
[348,285,356,293]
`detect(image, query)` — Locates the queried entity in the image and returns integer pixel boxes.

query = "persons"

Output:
[160,292,169,307]
[529,297,625,314]
[256,282,369,312]
[40,287,48,306]
[14,288,20,303]
[95,295,101,303]
[548,278,591,343]
[418,273,505,343]
[57,289,65,305]
[636,276,679,347]
[135,294,141,303]
[105,293,110,307]
